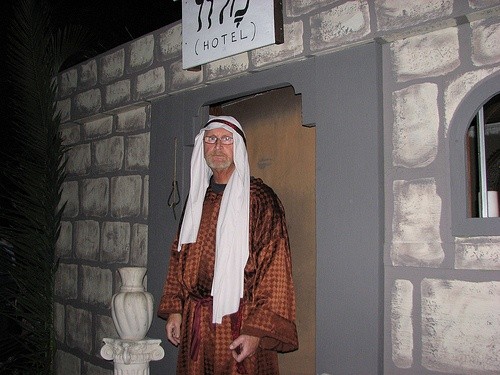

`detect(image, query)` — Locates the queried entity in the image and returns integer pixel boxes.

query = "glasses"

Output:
[203,135,234,145]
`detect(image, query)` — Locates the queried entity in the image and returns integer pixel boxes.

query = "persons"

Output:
[155,114,299,374]
